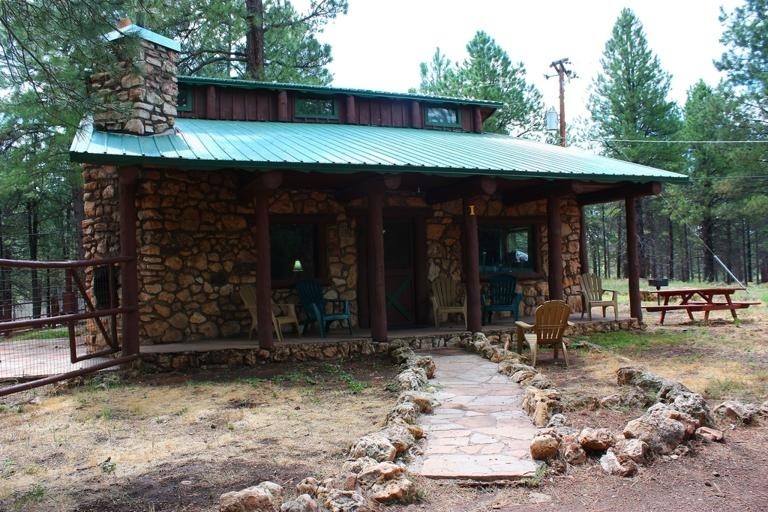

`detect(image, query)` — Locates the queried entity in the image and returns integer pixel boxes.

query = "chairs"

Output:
[299,279,354,339]
[514,298,571,368]
[430,274,469,329]
[577,272,621,322]
[480,273,522,322]
[237,285,301,343]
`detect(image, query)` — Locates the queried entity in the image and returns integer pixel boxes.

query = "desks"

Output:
[649,287,747,324]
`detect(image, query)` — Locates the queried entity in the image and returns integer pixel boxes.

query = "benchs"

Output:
[640,302,718,323]
[682,300,763,322]
